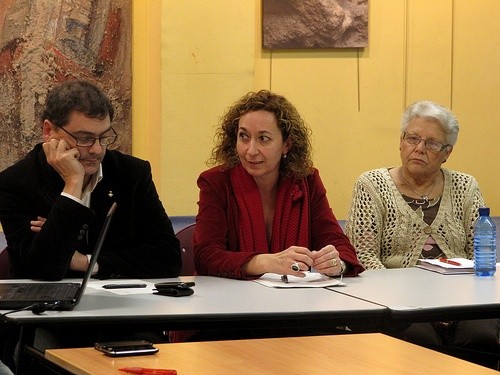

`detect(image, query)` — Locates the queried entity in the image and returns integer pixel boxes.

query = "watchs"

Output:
[339,260,346,275]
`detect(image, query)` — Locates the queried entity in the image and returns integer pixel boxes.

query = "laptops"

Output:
[0,202,117,311]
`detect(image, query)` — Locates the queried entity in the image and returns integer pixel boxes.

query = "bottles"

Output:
[474,208,496,276]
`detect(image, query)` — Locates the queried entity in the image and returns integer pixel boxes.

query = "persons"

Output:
[171,88,366,342]
[0,80,182,375]
[345,100,500,368]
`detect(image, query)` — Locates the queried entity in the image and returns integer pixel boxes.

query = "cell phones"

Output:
[96,340,159,357]
[154,281,195,288]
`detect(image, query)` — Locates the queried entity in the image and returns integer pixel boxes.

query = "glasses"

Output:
[51,120,118,147]
[403,132,449,153]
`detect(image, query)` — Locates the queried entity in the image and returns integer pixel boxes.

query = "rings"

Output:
[332,259,336,266]
[290,262,300,272]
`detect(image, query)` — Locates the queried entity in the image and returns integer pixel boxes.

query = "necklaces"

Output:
[407,180,433,200]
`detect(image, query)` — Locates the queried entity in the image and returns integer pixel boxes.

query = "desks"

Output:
[0,260,500,375]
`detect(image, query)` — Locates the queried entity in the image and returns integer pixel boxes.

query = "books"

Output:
[416,256,476,275]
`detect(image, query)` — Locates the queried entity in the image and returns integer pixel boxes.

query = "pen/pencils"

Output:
[102,283,147,289]
[119,367,177,375]
[281,275,287,283]
[439,257,461,266]
